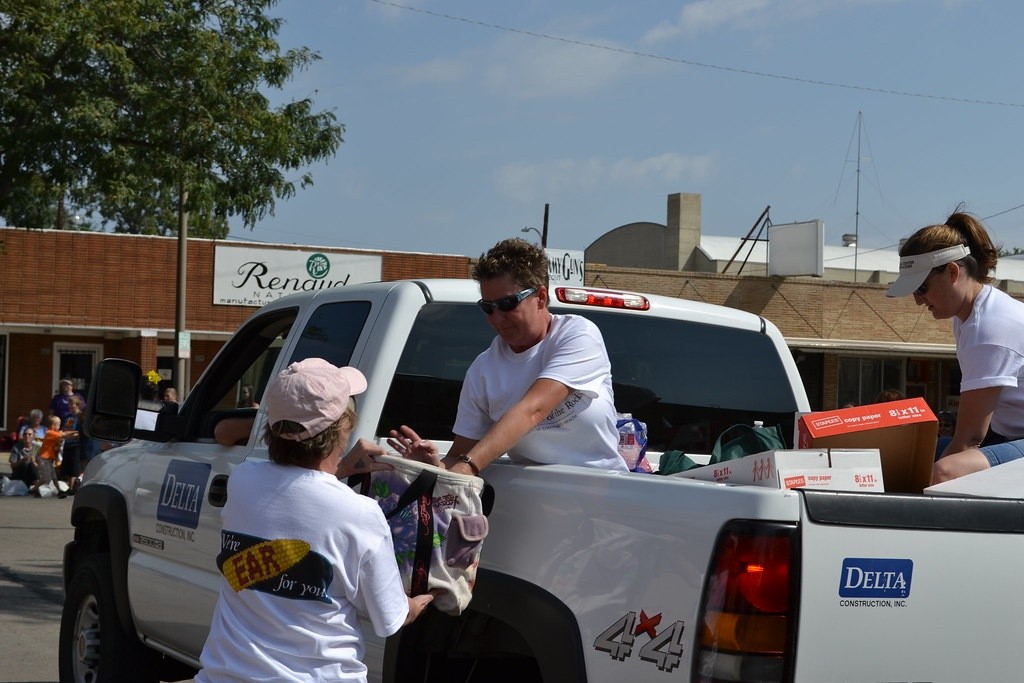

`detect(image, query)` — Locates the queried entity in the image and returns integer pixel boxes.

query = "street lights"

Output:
[169,78,237,415]
[520,203,550,248]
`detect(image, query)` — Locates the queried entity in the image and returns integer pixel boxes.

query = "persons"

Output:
[193,356,448,683]
[214,416,255,446]
[386,238,631,477]
[9,378,99,498]
[163,388,180,413]
[227,384,259,408]
[139,381,159,402]
[885,211,1024,488]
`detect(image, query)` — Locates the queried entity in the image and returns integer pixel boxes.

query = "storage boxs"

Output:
[668,447,886,494]
[799,397,939,494]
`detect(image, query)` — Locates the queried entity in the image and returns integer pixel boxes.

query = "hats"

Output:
[59,379,73,385]
[886,244,971,297]
[265,357,368,438]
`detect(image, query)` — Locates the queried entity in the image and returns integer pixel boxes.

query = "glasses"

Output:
[477,287,538,316]
[23,433,32,436]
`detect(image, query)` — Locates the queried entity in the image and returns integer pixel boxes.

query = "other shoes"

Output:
[28,488,41,497]
[65,488,76,496]
[57,490,67,498]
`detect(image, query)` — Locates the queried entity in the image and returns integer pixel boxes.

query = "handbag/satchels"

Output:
[615,412,652,473]
[338,453,490,617]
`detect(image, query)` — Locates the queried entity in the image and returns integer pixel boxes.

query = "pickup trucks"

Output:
[55,277,1024,683]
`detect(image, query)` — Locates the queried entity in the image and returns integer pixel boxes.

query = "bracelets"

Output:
[457,454,481,476]
